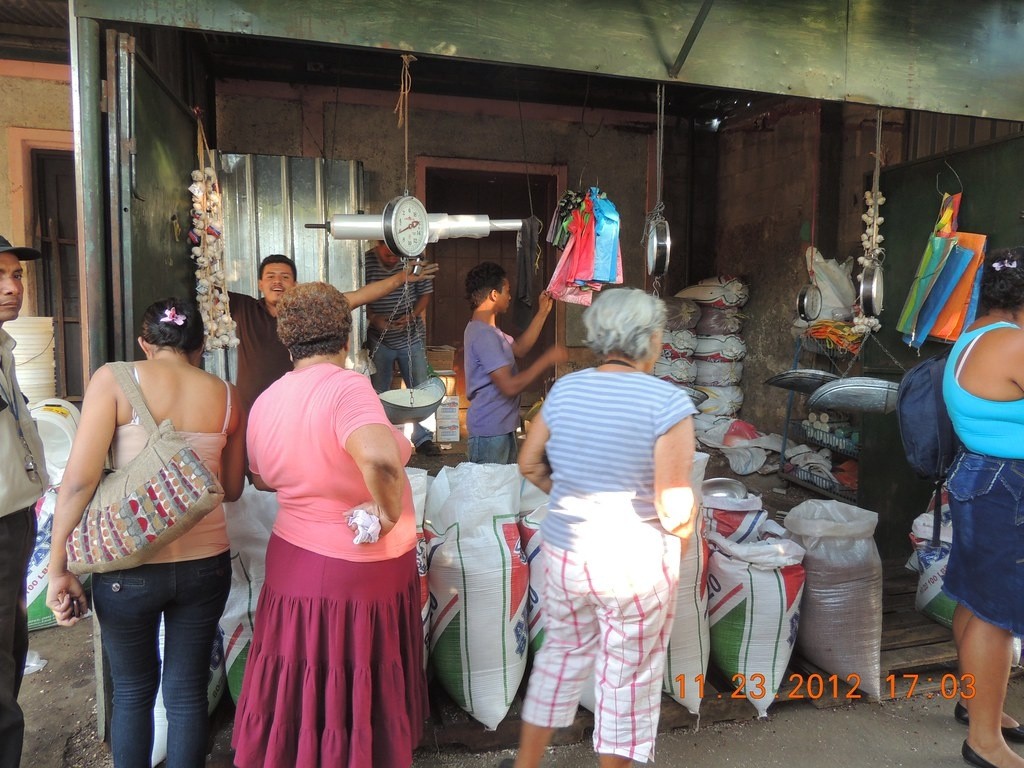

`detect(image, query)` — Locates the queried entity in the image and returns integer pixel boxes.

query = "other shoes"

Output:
[955,702,1024,744]
[961,739,997,768]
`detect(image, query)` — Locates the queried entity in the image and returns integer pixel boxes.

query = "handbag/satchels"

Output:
[63,362,225,576]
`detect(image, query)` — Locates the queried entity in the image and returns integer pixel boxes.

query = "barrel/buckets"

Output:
[2,316,57,409]
[29,398,81,486]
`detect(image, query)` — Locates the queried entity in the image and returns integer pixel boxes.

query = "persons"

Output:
[0,235,42,768]
[365,240,443,454]
[228,254,439,484]
[463,262,565,464]
[498,288,700,768]
[230,282,431,768]
[942,248,1023,768]
[45,300,244,768]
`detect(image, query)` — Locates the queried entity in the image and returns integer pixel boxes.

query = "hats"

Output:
[0,236,40,261]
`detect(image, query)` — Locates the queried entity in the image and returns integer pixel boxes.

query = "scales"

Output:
[647,215,709,407]
[764,283,843,393]
[804,247,907,418]
[362,196,446,424]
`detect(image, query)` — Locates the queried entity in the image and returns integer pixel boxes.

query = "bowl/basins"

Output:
[701,478,747,499]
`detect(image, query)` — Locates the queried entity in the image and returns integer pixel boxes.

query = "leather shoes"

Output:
[416,440,440,455]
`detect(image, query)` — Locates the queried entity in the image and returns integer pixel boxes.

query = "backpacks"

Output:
[897,346,957,475]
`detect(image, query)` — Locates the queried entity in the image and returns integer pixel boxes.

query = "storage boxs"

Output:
[391,347,460,443]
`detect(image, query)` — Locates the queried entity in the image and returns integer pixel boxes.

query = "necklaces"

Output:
[605,359,635,369]
